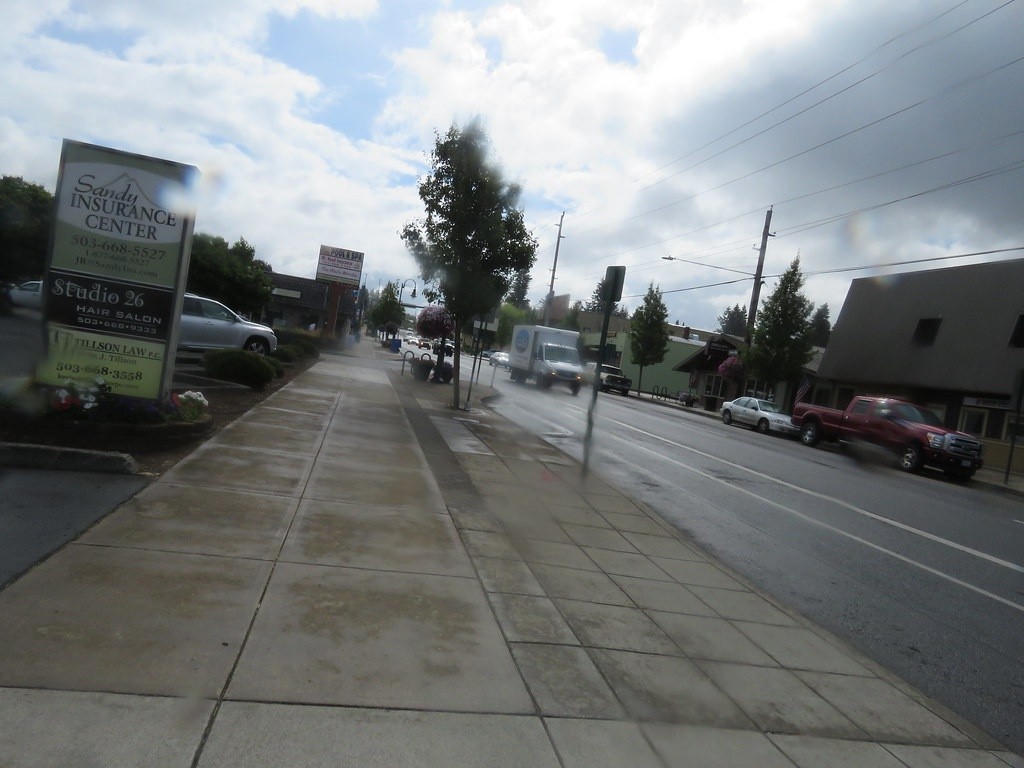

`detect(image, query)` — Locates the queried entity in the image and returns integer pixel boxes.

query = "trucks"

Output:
[585,362,632,395]
[508,324,584,396]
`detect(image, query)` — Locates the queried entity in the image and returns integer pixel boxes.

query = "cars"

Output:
[402,326,458,350]
[718,398,801,437]
[7,279,48,311]
[480,348,500,357]
[489,352,511,370]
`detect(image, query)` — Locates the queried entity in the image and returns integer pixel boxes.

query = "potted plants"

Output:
[679,393,701,407]
[408,358,435,381]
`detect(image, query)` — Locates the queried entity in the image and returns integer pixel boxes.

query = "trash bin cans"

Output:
[391,338,402,353]
[704,396,717,411]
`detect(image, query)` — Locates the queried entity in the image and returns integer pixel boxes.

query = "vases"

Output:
[441,375,453,384]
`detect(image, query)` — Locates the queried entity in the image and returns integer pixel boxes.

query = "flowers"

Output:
[439,363,455,376]
[417,305,454,340]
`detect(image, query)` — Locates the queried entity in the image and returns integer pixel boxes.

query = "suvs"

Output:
[174,293,280,366]
[435,338,453,356]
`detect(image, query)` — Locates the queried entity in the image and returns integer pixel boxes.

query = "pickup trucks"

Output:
[789,395,986,481]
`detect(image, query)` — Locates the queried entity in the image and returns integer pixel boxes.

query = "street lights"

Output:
[663,255,762,392]
[395,276,420,354]
